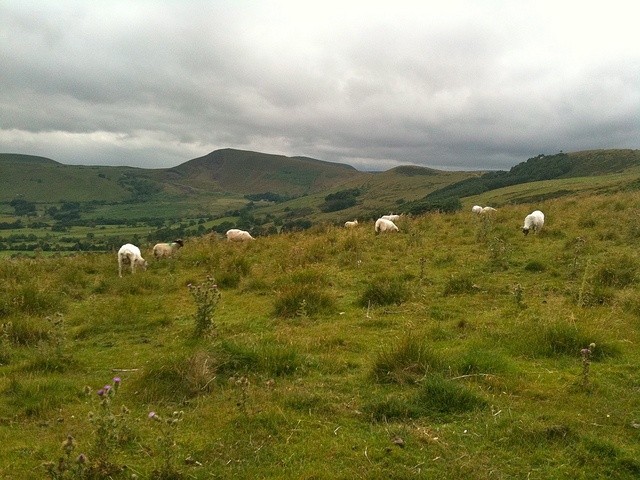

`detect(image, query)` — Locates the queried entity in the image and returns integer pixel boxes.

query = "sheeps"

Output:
[471,206,483,214]
[345,219,358,229]
[390,215,399,221]
[481,206,498,215]
[520,210,545,236]
[375,219,399,233]
[152,240,184,262]
[117,243,148,279]
[226,229,257,242]
[381,215,395,221]
